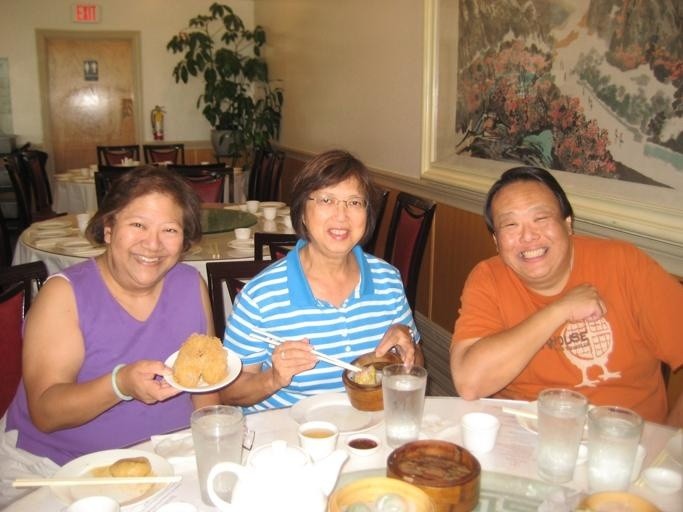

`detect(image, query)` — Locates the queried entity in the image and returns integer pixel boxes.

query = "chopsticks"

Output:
[260,331,362,373]
[11,477,171,488]
[627,426,681,494]
[501,405,537,418]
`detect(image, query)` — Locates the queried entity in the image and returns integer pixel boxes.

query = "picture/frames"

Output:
[412,1,681,243]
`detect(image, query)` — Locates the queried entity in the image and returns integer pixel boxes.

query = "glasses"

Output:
[307,193,369,212]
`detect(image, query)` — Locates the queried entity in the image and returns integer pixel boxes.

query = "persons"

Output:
[219,148,427,418]
[1,164,221,508]
[89,61,97,75]
[446,165,681,430]
[83,61,90,75]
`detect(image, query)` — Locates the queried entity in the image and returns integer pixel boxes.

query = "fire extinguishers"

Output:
[151,105,167,140]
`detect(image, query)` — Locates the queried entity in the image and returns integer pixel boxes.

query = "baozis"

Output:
[375,493,407,512]
[340,501,374,512]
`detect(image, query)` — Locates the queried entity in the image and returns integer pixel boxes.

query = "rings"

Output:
[280,350,285,359]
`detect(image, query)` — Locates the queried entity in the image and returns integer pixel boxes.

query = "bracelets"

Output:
[111,363,135,403]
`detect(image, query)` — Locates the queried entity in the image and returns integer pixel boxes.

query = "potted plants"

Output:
[163,2,290,170]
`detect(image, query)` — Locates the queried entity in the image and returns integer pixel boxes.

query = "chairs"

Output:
[660,279,682,392]
[206,233,300,344]
[383,191,437,318]
[0,142,286,234]
[1,260,48,416]
[361,186,390,257]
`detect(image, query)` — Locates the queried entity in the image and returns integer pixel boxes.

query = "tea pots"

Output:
[207,439,348,511]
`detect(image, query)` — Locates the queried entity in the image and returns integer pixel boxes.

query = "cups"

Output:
[76,213,90,235]
[296,421,381,477]
[533,384,644,492]
[380,361,428,446]
[64,495,121,512]
[646,430,683,511]
[463,412,497,458]
[190,404,244,507]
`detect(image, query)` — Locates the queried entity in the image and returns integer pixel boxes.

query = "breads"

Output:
[172,332,227,388]
[109,456,151,479]
[353,365,377,388]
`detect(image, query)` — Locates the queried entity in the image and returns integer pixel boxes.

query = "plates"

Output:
[223,199,291,258]
[518,399,595,444]
[48,450,179,510]
[289,391,382,435]
[164,343,240,392]
[30,221,106,258]
[54,162,96,182]
